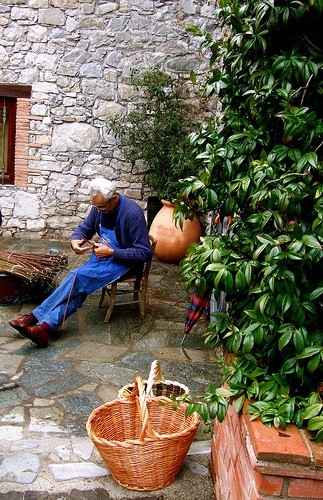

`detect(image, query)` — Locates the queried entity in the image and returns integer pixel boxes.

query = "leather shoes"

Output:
[9,312,39,337]
[18,322,51,347]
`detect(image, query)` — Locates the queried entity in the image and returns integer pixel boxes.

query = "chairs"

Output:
[99,234,157,323]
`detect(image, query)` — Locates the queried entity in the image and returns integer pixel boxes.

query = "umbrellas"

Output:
[180,213,232,348]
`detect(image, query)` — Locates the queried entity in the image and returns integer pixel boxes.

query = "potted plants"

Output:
[106,62,209,260]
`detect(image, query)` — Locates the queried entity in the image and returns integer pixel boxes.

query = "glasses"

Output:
[90,200,108,211]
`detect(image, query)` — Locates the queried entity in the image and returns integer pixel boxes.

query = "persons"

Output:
[9,179,152,347]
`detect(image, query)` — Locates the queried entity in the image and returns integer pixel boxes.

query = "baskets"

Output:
[85,377,201,491]
[118,360,189,401]
